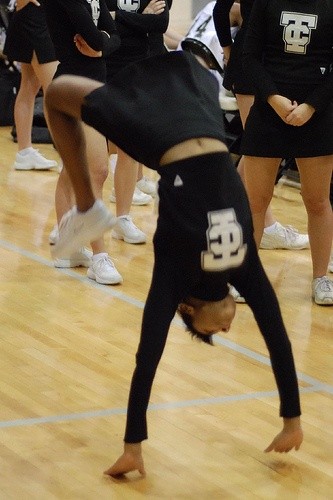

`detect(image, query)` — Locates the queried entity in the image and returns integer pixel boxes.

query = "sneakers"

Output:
[87,255,122,285]
[53,245,91,267]
[312,276,333,306]
[14,151,56,169]
[52,200,115,258]
[111,187,152,205]
[112,217,146,243]
[258,224,309,249]
[135,180,156,193]
[48,225,59,245]
[229,285,246,302]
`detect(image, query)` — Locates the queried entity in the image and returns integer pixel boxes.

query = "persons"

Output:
[43,50,303,478]
[0,0,333,308]
